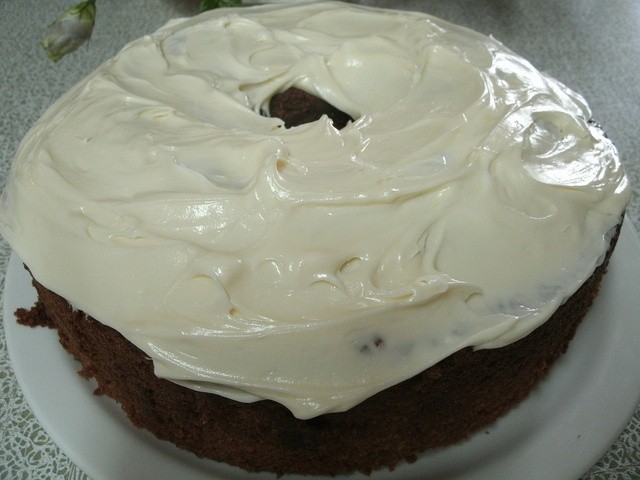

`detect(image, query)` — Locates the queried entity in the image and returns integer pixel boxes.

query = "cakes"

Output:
[3,2,628,472]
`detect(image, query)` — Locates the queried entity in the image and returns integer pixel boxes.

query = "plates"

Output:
[1,208,640,479]
